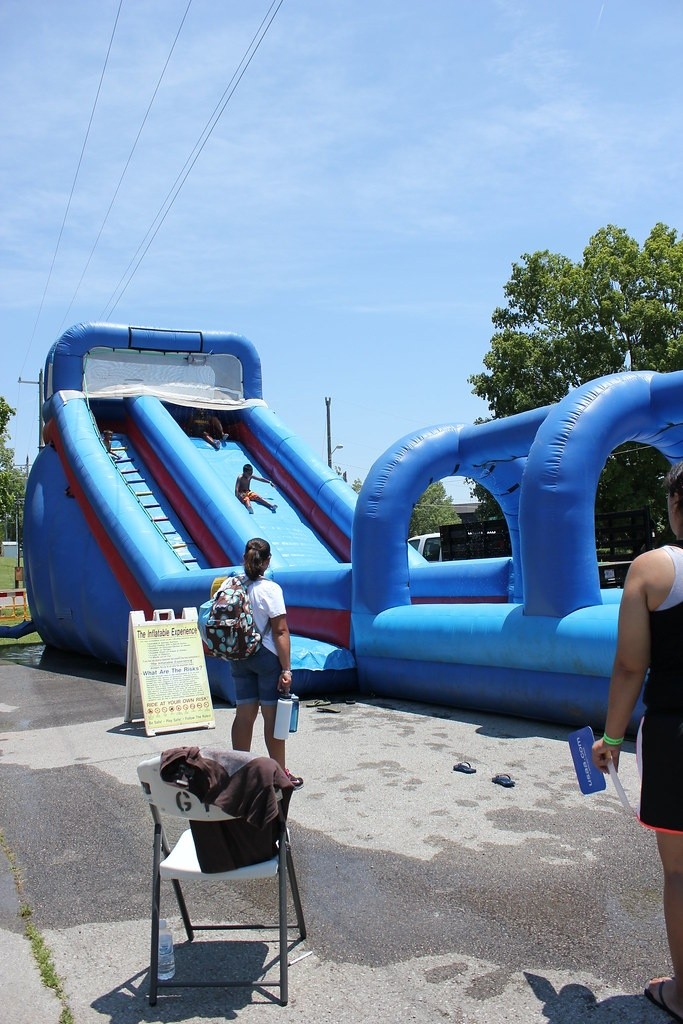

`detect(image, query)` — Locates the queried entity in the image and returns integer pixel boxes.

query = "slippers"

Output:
[305,696,331,706]
[346,696,355,704]
[453,762,476,774]
[644,974,683,1024]
[491,774,515,786]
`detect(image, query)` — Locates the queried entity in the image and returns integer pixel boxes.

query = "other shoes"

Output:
[317,708,341,713]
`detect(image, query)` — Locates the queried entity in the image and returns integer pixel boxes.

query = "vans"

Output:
[407,530,496,564]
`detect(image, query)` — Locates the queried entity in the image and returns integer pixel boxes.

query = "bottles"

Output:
[158,920,176,980]
[290,693,299,733]
[273,692,294,740]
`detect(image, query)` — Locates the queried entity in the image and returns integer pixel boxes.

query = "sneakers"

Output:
[283,767,304,790]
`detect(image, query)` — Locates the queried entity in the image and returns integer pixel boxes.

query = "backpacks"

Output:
[205,571,273,662]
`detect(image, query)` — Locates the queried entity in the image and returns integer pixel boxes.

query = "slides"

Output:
[42,390,511,652]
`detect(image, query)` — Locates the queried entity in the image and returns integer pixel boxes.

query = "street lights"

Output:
[326,443,344,469]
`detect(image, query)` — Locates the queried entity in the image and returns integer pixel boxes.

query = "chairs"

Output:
[138,748,307,1008]
[426,544,440,561]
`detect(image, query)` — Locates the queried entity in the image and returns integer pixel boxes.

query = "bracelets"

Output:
[603,733,624,745]
[282,669,292,675]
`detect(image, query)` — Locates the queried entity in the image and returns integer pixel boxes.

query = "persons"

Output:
[104,430,122,461]
[215,538,304,789]
[235,464,278,514]
[592,464,682,1021]
[188,412,227,448]
